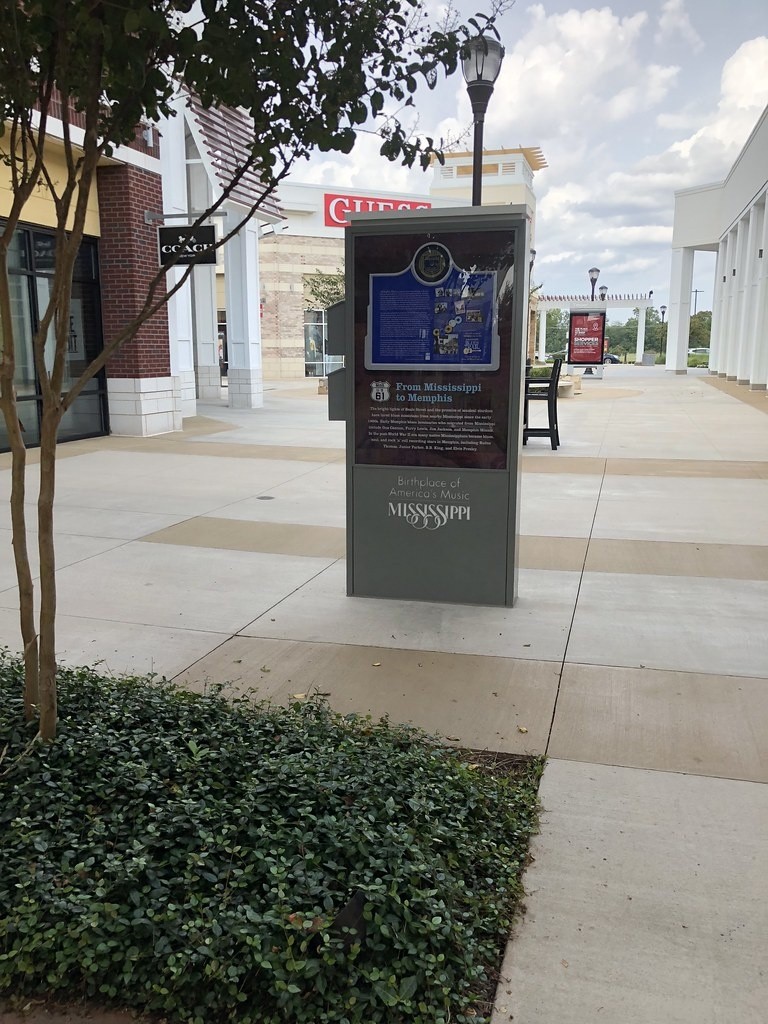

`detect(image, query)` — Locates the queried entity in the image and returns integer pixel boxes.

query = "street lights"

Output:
[660,306,667,353]
[588,268,600,302]
[460,35,505,206]
[599,286,608,301]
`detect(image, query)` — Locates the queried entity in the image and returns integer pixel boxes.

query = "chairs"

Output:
[523,359,563,450]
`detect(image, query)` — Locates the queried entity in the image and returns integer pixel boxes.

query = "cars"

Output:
[604,354,619,364]
[688,348,710,354]
[548,350,566,360]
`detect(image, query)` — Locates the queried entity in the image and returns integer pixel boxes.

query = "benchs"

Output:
[528,381,574,398]
